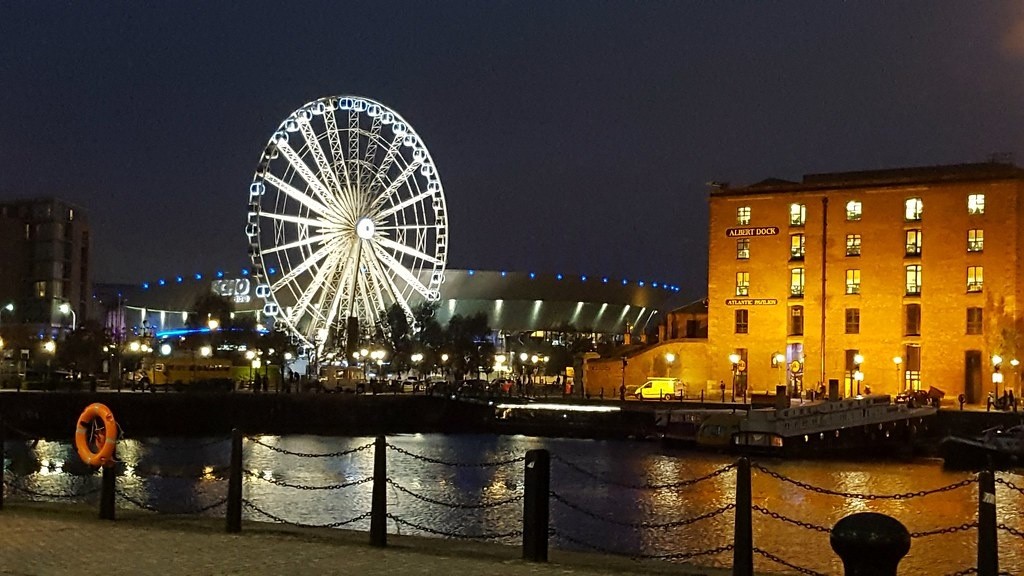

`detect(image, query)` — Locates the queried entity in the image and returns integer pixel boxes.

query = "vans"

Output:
[634,377,684,400]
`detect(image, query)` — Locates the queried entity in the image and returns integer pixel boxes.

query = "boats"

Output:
[942,424,1024,470]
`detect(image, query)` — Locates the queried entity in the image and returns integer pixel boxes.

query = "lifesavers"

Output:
[958,394,966,403]
[74,402,118,467]
[619,385,626,393]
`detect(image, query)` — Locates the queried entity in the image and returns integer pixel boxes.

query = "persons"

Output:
[368,378,378,392]
[557,376,561,383]
[856,393,862,398]
[988,389,1014,411]
[599,384,603,400]
[815,383,826,398]
[620,385,626,400]
[908,390,938,407]
[719,380,725,401]
[515,377,533,389]
[491,377,513,397]
[288,370,300,382]
[462,381,469,387]
[253,374,268,392]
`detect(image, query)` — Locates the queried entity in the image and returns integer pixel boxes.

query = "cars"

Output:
[894,389,930,405]
[424,375,510,396]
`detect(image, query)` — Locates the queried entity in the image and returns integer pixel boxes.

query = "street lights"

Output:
[360,349,368,394]
[894,357,902,395]
[993,355,1002,399]
[162,344,171,393]
[59,304,76,331]
[777,354,785,385]
[854,354,864,395]
[246,350,255,390]
[130,341,139,391]
[441,354,448,377]
[729,354,740,402]
